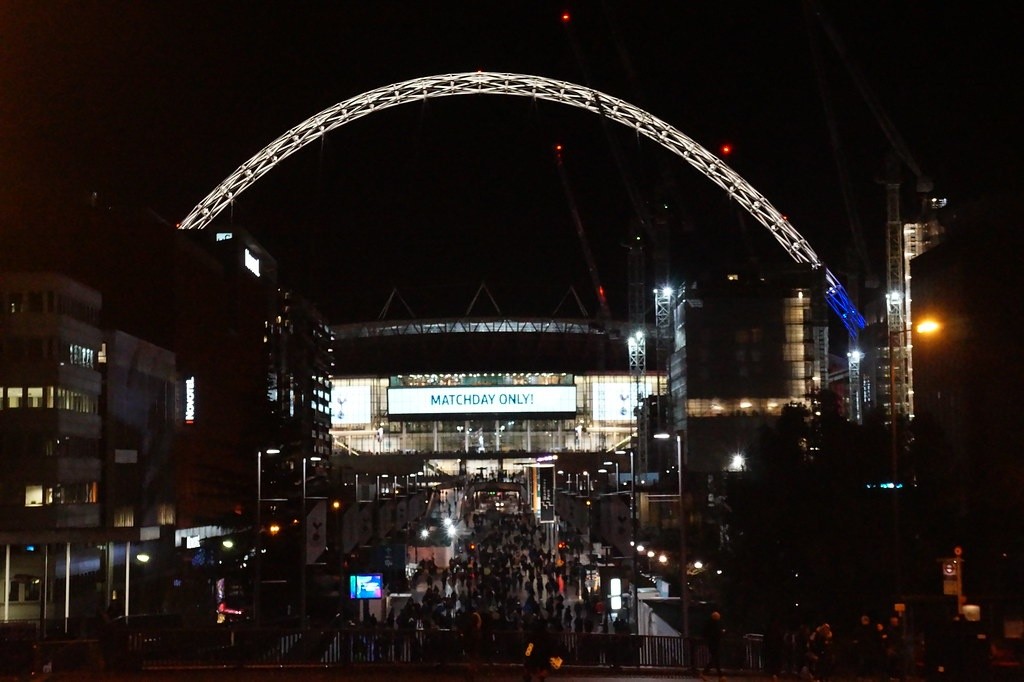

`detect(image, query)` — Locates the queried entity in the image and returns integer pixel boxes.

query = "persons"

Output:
[701,611,913,682]
[370,474,594,664]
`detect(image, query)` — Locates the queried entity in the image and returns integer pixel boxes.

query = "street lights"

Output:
[651,429,690,639]
[885,316,945,600]
[406,473,417,495]
[603,459,621,492]
[300,454,331,627]
[615,450,639,633]
[376,474,389,501]
[583,471,591,497]
[254,446,282,614]
[415,470,424,490]
